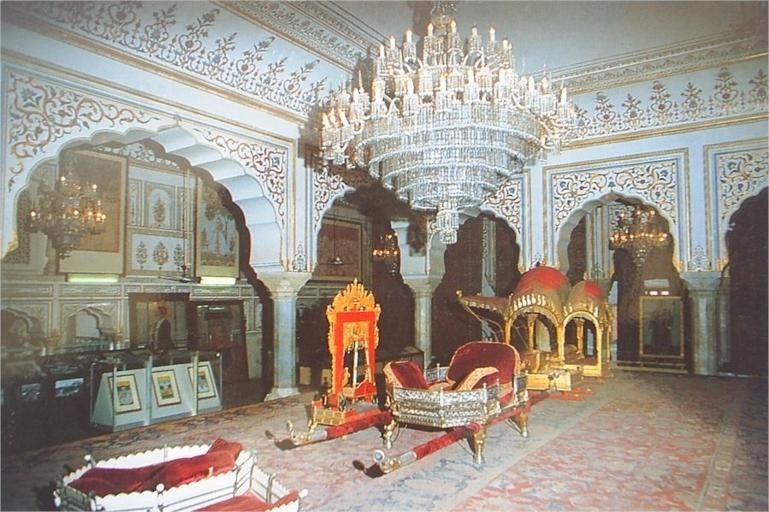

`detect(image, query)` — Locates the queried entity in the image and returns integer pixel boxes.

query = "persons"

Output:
[151,297,180,356]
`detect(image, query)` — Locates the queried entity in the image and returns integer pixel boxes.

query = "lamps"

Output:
[25,176,106,260]
[372,232,400,274]
[609,206,668,268]
[319,0,571,244]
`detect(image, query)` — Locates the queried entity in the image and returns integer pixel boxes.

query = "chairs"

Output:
[383,341,528,465]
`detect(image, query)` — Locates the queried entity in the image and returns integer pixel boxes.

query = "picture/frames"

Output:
[109,374,142,414]
[195,177,242,280]
[151,369,181,408]
[309,217,364,284]
[187,365,216,400]
[639,296,684,359]
[56,148,127,276]
